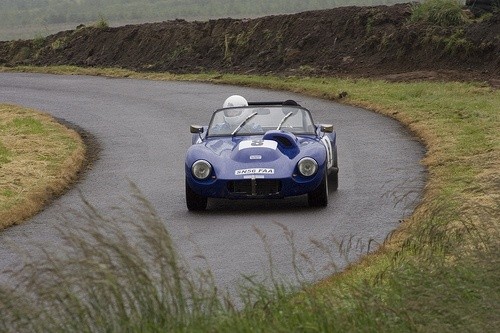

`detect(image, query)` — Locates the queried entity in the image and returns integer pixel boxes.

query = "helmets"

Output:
[222,95,249,128]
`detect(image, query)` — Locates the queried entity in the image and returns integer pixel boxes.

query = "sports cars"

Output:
[184,101,339,211]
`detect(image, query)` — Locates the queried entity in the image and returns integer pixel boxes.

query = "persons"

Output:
[209,95,263,134]
